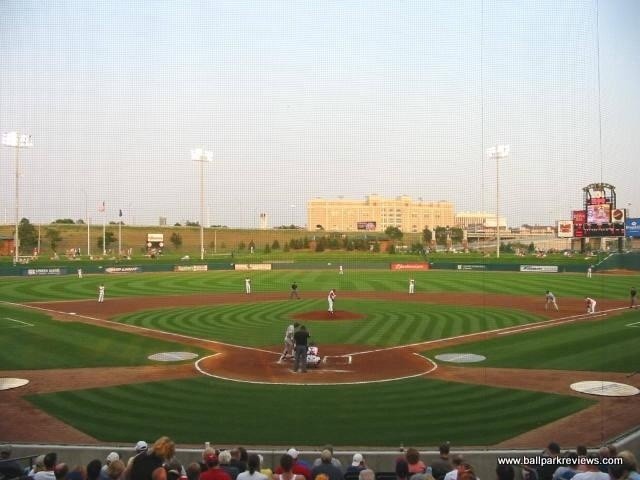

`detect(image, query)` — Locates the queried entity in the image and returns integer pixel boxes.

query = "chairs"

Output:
[344,471,363,480]
[374,470,396,479]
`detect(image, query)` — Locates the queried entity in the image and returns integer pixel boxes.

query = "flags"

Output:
[99,201,106,212]
[118,209,123,217]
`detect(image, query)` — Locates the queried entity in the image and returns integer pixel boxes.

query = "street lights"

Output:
[487,143,509,258]
[2,131,36,263]
[288,204,296,228]
[190,146,214,260]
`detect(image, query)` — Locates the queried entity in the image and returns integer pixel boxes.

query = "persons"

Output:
[291,324,311,373]
[630,286,638,310]
[586,267,592,280]
[244,277,251,295]
[97,283,105,303]
[584,296,597,314]
[306,343,321,369]
[10,247,162,264]
[409,277,415,293]
[544,290,559,312]
[77,267,82,279]
[328,288,336,315]
[338,264,343,274]
[1,435,639,479]
[275,321,300,366]
[290,281,300,299]
